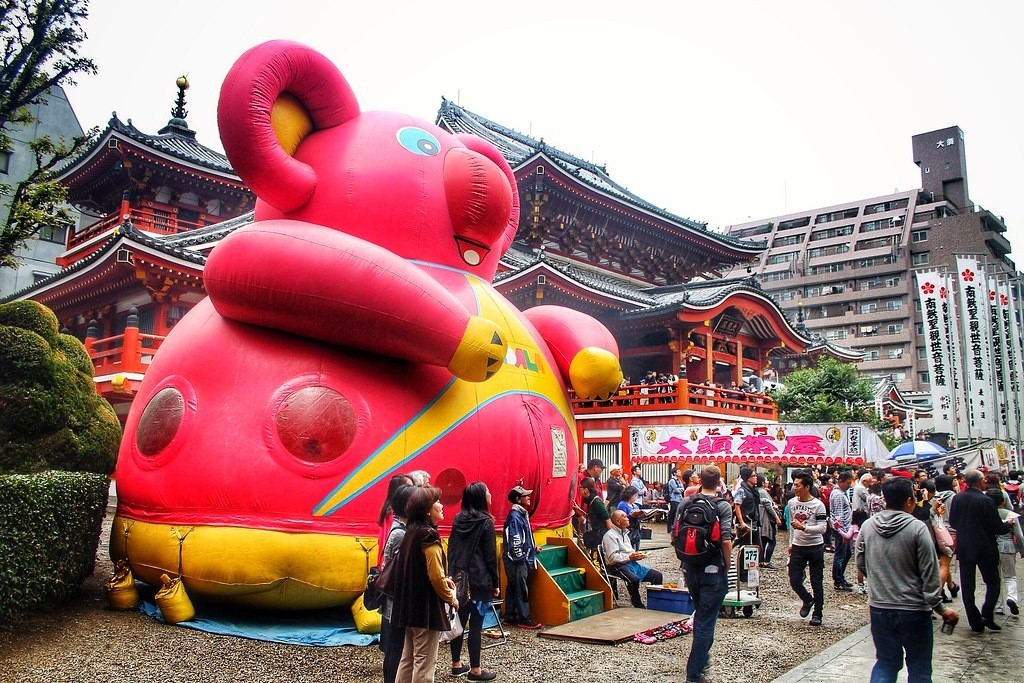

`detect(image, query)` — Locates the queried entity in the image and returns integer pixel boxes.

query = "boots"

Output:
[940,589,952,602]
[949,582,959,597]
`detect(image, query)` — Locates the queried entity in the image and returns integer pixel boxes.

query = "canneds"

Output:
[941,619,955,635]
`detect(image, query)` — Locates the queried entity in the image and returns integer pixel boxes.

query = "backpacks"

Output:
[674,494,727,564]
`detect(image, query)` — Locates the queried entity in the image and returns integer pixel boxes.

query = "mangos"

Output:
[663,584,677,588]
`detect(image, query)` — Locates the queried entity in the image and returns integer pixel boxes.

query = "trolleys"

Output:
[722,544,761,619]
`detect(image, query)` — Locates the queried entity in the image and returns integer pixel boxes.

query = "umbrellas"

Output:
[887,441,948,469]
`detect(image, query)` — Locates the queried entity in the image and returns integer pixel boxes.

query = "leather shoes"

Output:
[834,581,853,592]
[800,598,813,617]
[810,614,821,625]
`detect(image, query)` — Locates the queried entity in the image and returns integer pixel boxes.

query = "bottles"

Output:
[942,618,956,635]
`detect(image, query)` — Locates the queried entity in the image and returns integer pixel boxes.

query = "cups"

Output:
[671,582,678,588]
[663,582,670,588]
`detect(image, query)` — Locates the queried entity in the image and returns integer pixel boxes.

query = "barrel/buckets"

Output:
[640,524,652,539]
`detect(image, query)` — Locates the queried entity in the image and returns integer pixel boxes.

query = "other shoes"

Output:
[1007,599,1019,615]
[982,616,1002,632]
[995,608,1004,615]
[972,623,984,632]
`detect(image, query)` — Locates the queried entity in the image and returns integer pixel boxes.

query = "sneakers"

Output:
[503,615,542,628]
[762,563,778,570]
[858,586,869,594]
[452,662,471,676]
[466,670,496,682]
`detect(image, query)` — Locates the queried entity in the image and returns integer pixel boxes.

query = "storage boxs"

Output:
[646,587,696,616]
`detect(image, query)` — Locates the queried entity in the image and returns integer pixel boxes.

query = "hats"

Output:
[740,468,753,481]
[590,459,607,470]
[682,468,696,482]
[610,464,623,472]
[508,485,533,503]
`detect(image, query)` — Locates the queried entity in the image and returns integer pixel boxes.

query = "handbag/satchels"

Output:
[363,566,383,609]
[933,515,953,549]
[453,571,471,606]
[436,586,463,642]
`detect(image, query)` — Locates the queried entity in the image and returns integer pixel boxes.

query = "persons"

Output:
[572,459,699,564]
[618,370,770,412]
[895,422,911,441]
[949,441,956,449]
[672,464,732,683]
[447,481,500,683]
[378,469,460,683]
[503,485,541,628]
[602,510,662,609]
[916,432,928,441]
[887,413,895,427]
[719,464,1024,631]
[855,475,959,683]
